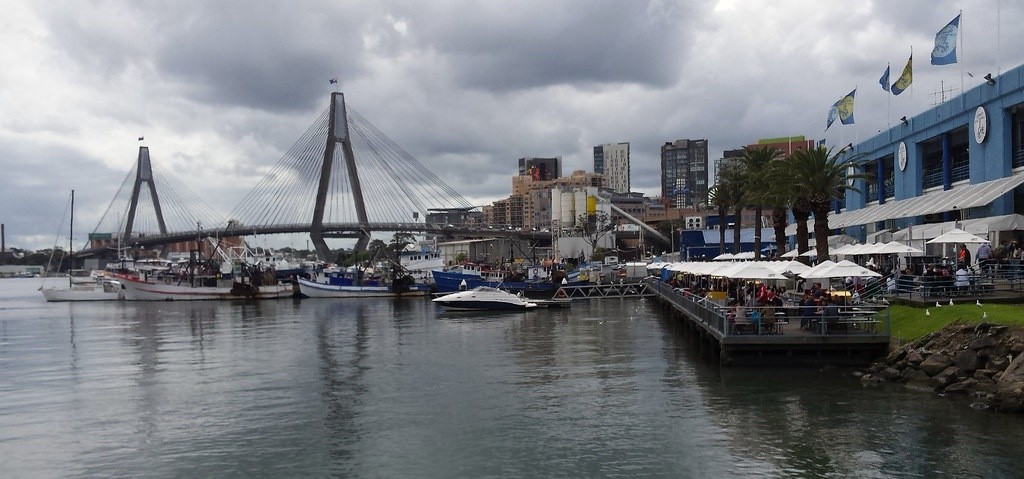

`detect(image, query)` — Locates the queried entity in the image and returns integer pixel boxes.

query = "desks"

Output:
[815,310,878,332]
[726,312,786,333]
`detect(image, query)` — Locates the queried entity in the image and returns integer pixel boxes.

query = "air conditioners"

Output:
[714,225,720,230]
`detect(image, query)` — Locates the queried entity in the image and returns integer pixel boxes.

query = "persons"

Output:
[973,241,994,269]
[722,279,840,335]
[924,261,975,296]
[994,240,1009,264]
[1010,241,1023,260]
[955,244,972,270]
[670,277,708,299]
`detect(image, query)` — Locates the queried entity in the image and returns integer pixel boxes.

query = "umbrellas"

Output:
[780,242,924,257]
[646,260,729,276]
[926,228,990,243]
[730,259,882,278]
[714,252,766,260]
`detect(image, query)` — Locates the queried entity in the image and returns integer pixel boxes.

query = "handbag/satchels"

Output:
[726,315,736,323]
[751,310,760,323]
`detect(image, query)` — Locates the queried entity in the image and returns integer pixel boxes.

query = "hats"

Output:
[959,243,966,247]
[755,280,764,284]
[673,277,676,280]
[804,289,812,293]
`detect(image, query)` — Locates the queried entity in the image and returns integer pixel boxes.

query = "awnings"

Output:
[796,213,1024,246]
[785,172,1024,235]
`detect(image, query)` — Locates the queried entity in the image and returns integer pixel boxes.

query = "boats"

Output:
[35,189,427,304]
[430,261,606,297]
[431,284,537,312]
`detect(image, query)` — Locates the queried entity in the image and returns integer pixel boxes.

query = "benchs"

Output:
[812,316,883,335]
[728,322,788,335]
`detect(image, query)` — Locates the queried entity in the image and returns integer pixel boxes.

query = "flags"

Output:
[930,13,962,65]
[879,65,891,92]
[825,89,856,132]
[891,54,913,96]
